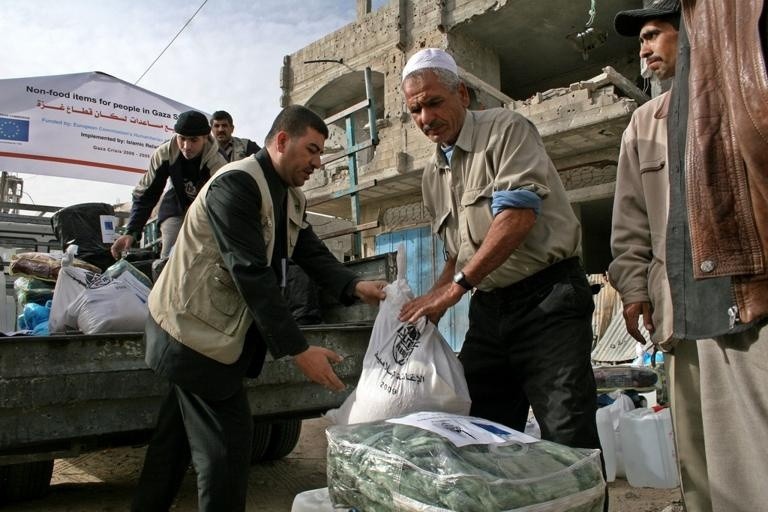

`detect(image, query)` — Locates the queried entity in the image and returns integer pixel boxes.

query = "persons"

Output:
[208,109,262,162]
[397,49,607,511]
[607,3,707,510]
[669,3,767,511]
[112,112,230,287]
[134,106,391,510]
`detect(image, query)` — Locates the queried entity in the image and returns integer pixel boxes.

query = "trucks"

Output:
[0,170,400,501]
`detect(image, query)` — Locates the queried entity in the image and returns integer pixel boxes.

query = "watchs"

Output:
[453,272,474,291]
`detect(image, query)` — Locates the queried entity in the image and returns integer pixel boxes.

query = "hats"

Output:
[615,2,683,37]
[175,111,211,136]
[402,49,458,81]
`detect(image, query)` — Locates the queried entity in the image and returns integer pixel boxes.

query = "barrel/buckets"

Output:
[619,406,680,488]
[596,394,635,483]
